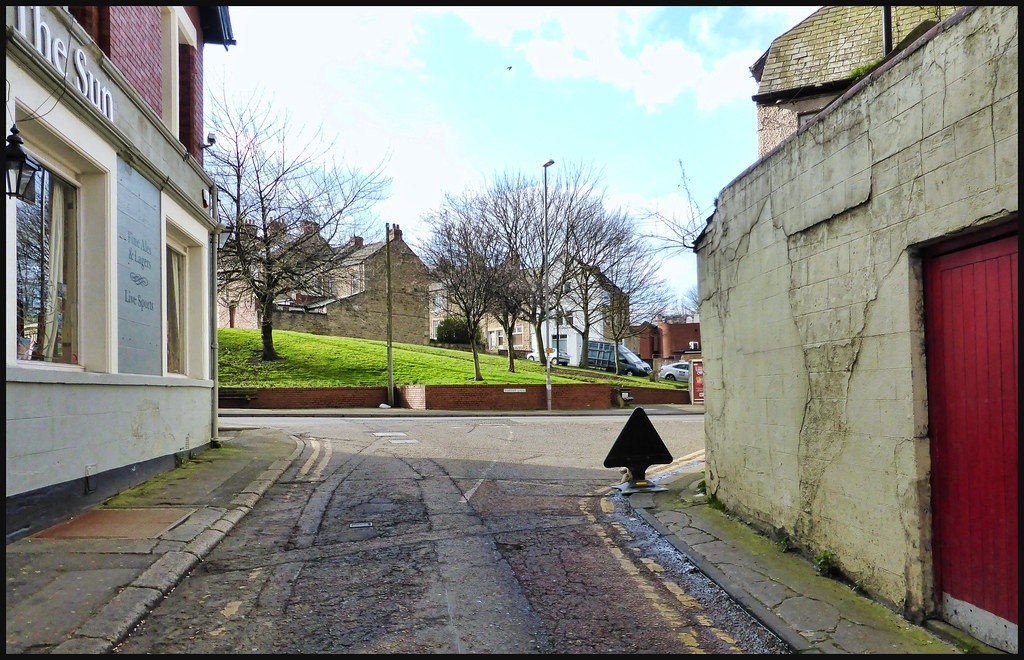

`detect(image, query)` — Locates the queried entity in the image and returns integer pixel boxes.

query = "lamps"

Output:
[5,124,43,206]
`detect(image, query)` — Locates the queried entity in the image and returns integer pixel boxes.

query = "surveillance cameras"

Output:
[207,133,216,144]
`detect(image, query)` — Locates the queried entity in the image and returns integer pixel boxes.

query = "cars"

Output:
[658,361,690,382]
[527,348,571,366]
[579,340,651,377]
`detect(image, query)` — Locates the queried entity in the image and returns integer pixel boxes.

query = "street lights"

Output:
[542,158,555,413]
